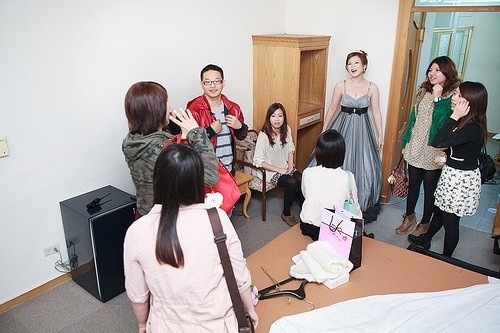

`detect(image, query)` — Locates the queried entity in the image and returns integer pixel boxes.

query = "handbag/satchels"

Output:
[319,206,361,273]
[238,314,254,333]
[478,141,496,183]
[388,155,409,197]
[163,132,241,213]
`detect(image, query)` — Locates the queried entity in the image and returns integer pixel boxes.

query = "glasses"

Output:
[203,80,223,86]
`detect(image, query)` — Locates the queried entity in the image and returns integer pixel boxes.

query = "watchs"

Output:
[433,96,442,102]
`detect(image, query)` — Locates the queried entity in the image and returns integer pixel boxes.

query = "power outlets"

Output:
[43,243,59,256]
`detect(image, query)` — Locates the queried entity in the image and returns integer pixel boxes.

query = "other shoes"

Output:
[281,213,298,227]
[407,234,431,249]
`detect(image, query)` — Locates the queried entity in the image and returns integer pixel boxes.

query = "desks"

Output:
[233,171,254,219]
[59,185,138,303]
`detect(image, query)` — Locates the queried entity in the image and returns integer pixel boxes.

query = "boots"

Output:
[396,212,416,234]
[411,222,430,236]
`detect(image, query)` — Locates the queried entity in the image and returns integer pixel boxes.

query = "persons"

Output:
[307,50,383,224]
[185,64,249,178]
[124,143,259,333]
[252,103,305,227]
[122,81,220,220]
[396,56,462,236]
[408,81,488,257]
[300,129,375,242]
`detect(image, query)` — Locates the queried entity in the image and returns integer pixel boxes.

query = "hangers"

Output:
[255,273,315,311]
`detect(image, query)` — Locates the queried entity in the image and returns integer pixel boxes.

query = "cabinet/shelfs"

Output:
[252,33,332,198]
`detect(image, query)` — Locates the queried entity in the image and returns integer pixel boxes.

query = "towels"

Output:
[288,240,355,289]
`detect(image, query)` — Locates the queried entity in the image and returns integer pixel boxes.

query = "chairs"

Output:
[235,129,276,221]
